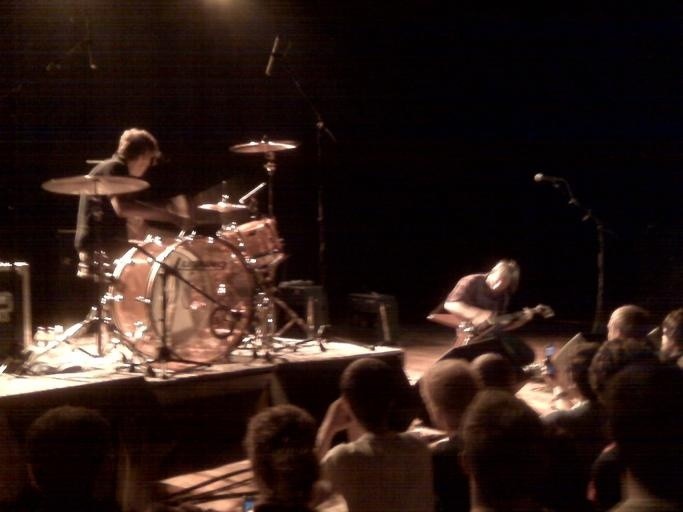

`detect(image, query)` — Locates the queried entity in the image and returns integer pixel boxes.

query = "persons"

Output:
[71,129,199,280]
[235,403,344,510]
[312,357,438,507]
[444,259,537,375]
[23,404,131,511]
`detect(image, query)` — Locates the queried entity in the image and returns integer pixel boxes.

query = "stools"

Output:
[72,263,127,369]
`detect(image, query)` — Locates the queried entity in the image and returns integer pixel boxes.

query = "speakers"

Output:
[406,336,526,431]
[547,333,607,396]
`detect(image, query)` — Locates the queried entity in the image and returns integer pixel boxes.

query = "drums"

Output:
[216,218,282,266]
[109,235,254,370]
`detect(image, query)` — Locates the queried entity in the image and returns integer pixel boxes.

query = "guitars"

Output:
[428,304,555,348]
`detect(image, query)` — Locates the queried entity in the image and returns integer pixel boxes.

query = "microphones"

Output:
[533,172,564,185]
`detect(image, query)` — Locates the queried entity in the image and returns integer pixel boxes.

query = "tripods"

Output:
[18,208,156,378]
[114,247,232,378]
[231,171,322,360]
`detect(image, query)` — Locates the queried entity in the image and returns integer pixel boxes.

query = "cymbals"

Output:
[86,160,105,164]
[230,140,298,153]
[197,202,247,212]
[44,176,149,195]
